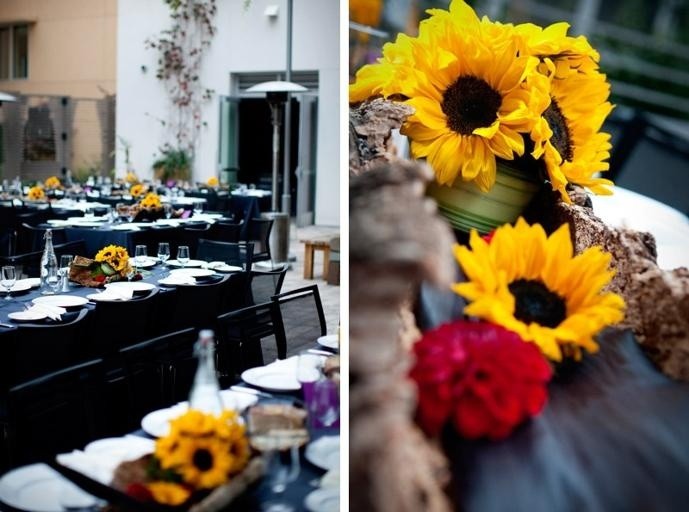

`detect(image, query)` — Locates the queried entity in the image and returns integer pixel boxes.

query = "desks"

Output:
[0,177,340,512]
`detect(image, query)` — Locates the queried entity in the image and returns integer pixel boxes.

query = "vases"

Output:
[420,153,534,237]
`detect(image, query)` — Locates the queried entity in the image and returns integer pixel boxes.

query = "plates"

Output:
[47,216,188,231]
[0,335,339,510]
[0,255,241,323]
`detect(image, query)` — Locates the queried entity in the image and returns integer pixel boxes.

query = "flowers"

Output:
[349,0,615,206]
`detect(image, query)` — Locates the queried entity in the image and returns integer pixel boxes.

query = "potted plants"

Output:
[150,142,190,182]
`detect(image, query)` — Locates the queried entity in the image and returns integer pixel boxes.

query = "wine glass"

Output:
[192,202,204,213]
[157,243,170,262]
[135,245,147,268]
[176,246,189,268]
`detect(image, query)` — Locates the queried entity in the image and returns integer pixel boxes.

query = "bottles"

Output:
[39,228,59,294]
